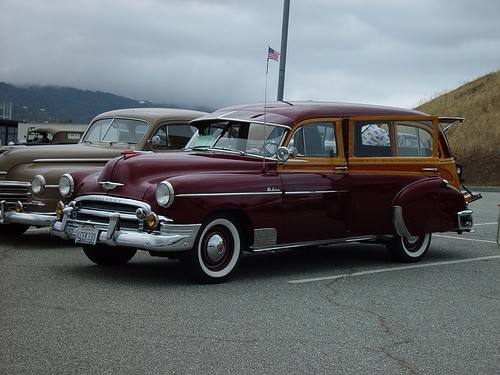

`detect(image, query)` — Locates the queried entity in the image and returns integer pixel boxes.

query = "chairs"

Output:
[118,125,428,157]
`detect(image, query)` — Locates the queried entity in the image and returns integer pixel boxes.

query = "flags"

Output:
[268,47,280,63]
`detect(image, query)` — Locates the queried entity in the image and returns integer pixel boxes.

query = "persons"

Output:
[36,132,50,144]
[361,122,391,146]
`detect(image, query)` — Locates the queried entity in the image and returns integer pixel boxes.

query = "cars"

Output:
[0,105,212,255]
[53,95,482,284]
